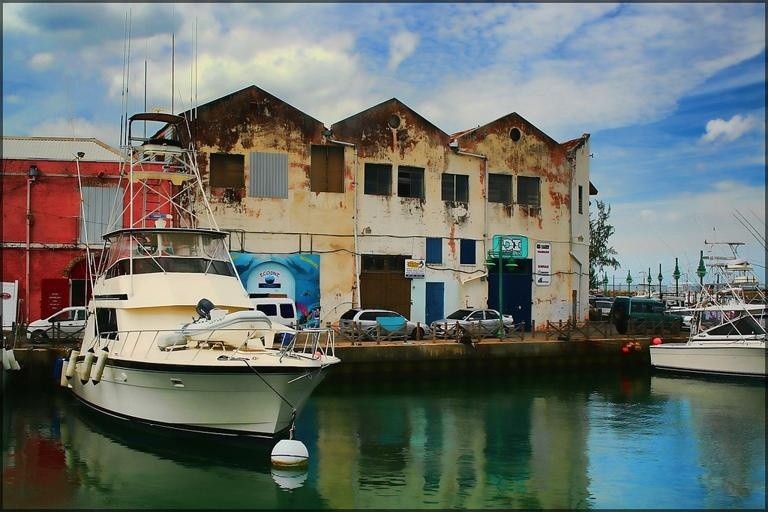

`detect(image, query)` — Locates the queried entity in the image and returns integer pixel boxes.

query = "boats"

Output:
[648,369,767,499]
[648,209,766,375]
[51,390,333,511]
[51,9,341,448]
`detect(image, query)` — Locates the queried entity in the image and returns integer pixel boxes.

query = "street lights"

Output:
[694,248,707,301]
[646,267,653,297]
[625,269,631,296]
[671,257,681,304]
[485,235,519,341]
[602,271,608,296]
[657,263,663,297]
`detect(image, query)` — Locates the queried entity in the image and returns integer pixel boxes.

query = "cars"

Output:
[26,305,89,344]
[430,308,515,336]
[587,295,696,335]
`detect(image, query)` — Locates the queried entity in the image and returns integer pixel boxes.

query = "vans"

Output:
[250,297,297,332]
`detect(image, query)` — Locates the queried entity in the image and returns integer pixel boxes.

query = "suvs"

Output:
[339,307,430,340]
